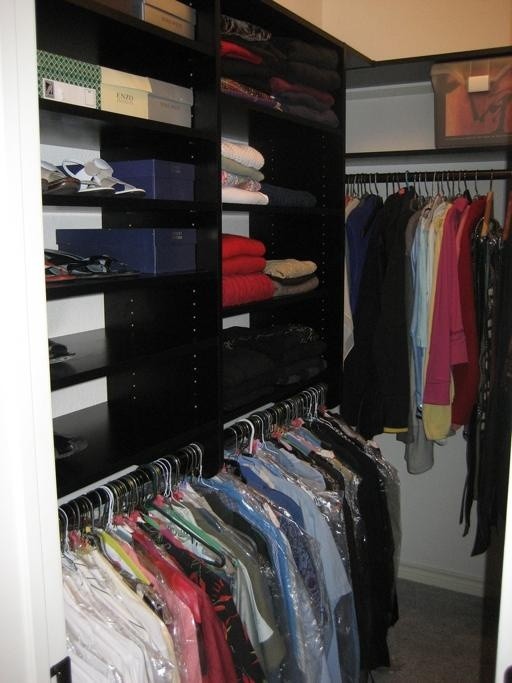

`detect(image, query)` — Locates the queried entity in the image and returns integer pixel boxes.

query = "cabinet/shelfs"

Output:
[35,1,346,534]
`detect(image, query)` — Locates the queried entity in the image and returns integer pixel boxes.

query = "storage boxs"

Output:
[36,1,198,128]
[53,224,198,274]
[100,155,196,201]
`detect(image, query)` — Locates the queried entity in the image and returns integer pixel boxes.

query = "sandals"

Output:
[62,157,145,199]
[48,339,77,365]
[45,262,76,282]
[46,248,141,283]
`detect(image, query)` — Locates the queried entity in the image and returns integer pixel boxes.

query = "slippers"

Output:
[42,167,81,198]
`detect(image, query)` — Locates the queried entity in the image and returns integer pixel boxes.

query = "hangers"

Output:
[344,168,511,245]
